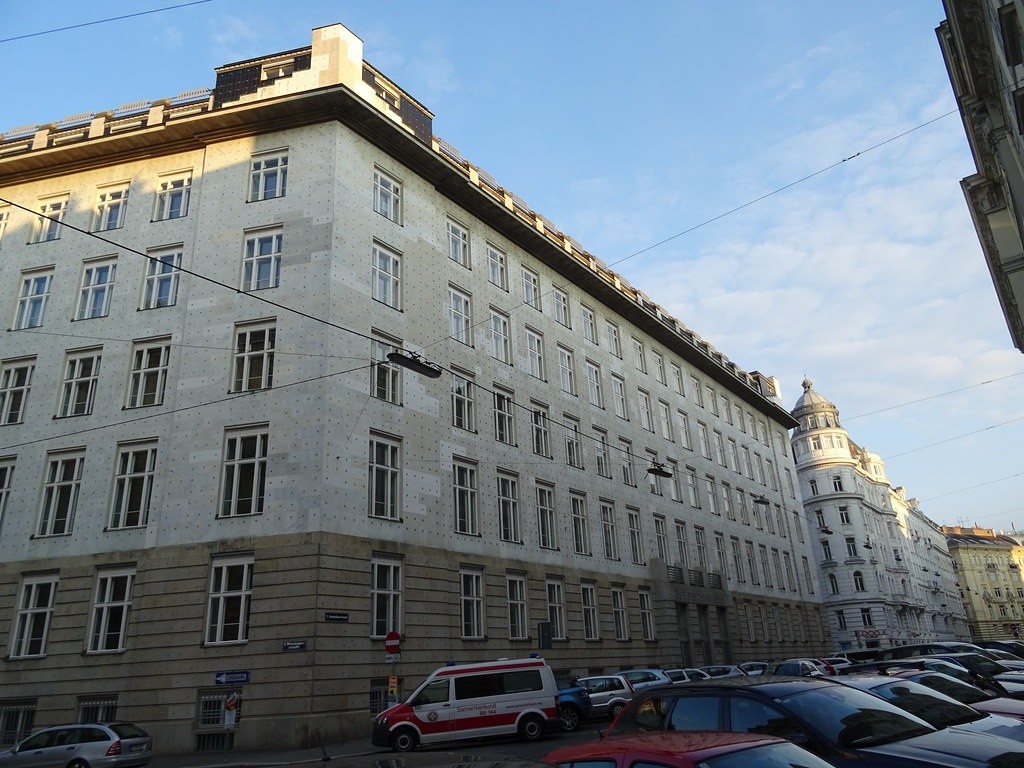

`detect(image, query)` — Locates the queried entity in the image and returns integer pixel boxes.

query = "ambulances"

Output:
[370,653,563,754]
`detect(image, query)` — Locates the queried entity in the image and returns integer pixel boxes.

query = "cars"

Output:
[543,730,835,767]
[665,668,712,697]
[615,678,1024,768]
[577,675,636,726]
[759,659,823,679]
[698,664,749,682]
[540,674,594,733]
[813,673,1024,745]
[811,637,1024,700]
[738,662,770,678]
[596,668,675,704]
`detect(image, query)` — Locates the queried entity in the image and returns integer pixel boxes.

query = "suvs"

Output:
[0,720,155,768]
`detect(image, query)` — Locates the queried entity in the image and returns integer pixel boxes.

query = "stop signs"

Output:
[385,631,401,655]
